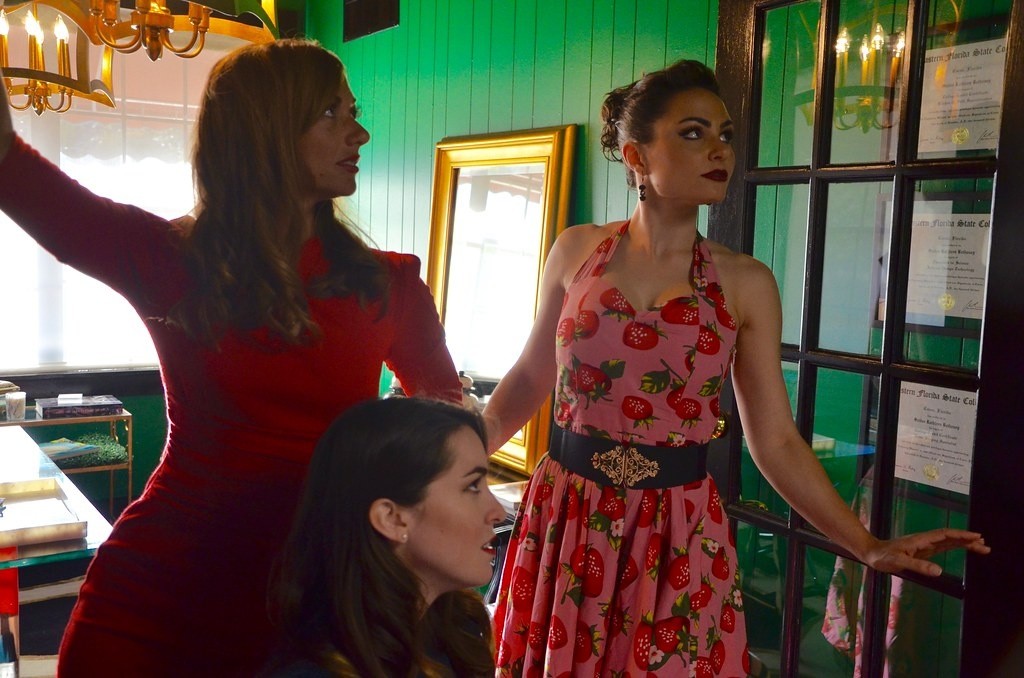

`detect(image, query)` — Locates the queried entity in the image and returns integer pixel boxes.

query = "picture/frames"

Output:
[425,124,577,482]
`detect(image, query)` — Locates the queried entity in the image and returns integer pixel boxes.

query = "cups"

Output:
[6,392,26,422]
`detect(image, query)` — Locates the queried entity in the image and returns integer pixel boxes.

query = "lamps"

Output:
[813,0,960,134]
[0,0,214,117]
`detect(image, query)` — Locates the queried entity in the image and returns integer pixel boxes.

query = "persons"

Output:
[258,395,497,678]
[820,463,930,678]
[0,36,463,678]
[479,58,992,678]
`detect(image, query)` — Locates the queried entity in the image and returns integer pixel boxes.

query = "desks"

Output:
[0,408,132,678]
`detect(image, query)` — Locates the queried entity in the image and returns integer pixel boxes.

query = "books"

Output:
[34,393,124,465]
